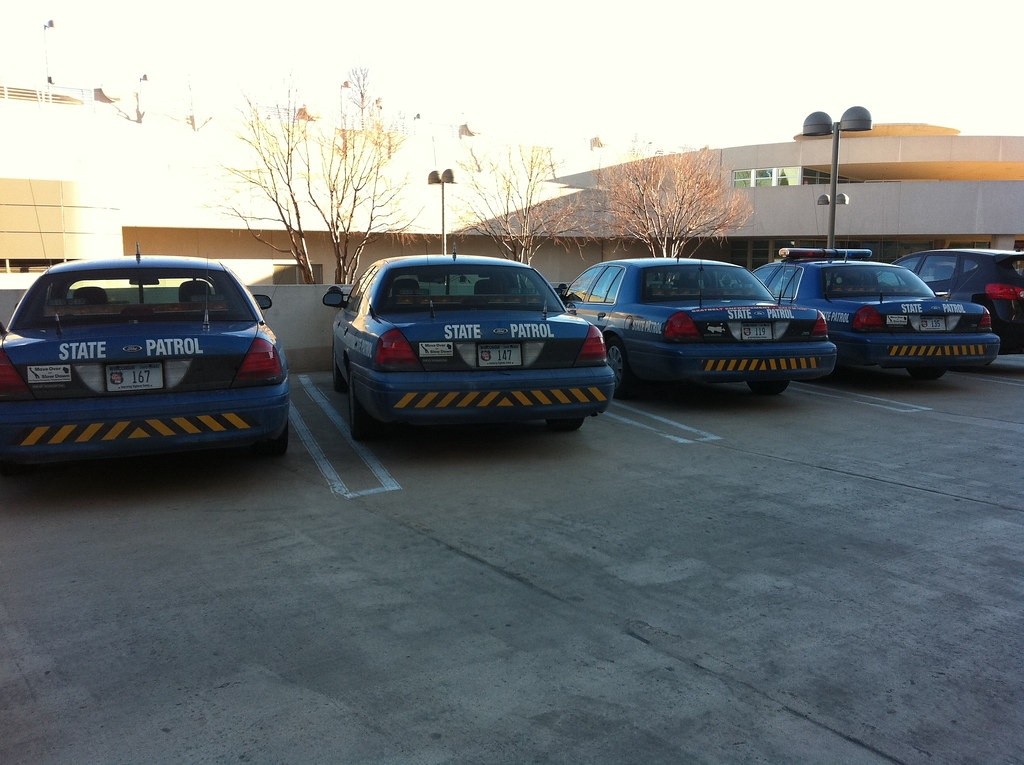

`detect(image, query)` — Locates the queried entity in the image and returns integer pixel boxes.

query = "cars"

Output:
[558,258,837,399]
[0,255,290,473]
[323,254,616,442]
[722,248,1000,383]
[869,250,1024,373]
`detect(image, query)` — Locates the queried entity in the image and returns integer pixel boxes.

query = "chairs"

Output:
[73,280,214,306]
[935,265,954,280]
[391,278,502,295]
[677,274,700,294]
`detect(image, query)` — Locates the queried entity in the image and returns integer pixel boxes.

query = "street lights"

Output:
[429,169,456,255]
[803,107,872,248]
